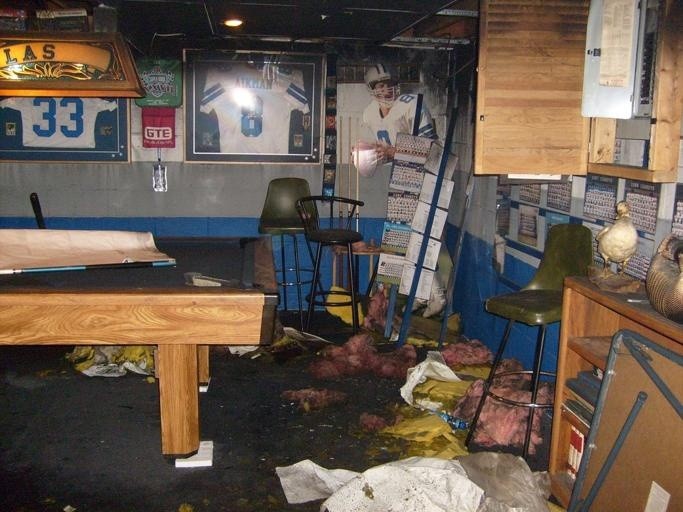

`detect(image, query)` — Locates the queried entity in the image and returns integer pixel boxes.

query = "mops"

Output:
[326,251,366,328]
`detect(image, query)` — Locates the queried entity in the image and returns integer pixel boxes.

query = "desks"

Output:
[0,234,282,460]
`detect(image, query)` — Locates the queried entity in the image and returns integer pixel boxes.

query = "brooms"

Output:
[269,331,307,367]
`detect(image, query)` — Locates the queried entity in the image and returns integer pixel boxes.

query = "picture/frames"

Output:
[180,47,325,167]
[0,97,132,165]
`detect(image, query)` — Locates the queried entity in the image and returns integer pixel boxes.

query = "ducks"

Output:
[595,199,639,275]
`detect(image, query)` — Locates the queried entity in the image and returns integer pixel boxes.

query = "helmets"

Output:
[363,61,402,109]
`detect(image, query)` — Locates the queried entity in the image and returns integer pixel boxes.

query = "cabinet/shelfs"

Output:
[546,274,683,508]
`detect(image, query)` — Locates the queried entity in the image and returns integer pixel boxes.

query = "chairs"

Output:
[461,223,594,460]
[257,176,363,339]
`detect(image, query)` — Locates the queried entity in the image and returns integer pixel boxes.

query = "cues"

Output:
[333,116,359,297]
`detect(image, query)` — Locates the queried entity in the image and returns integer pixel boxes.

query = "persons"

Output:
[350,60,441,171]
